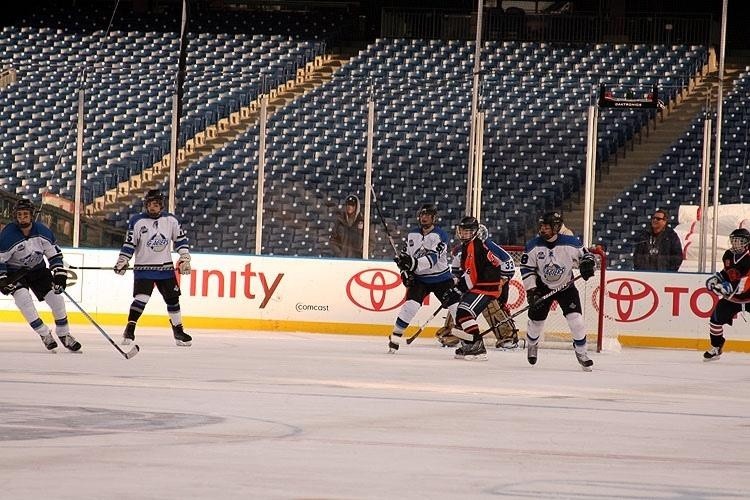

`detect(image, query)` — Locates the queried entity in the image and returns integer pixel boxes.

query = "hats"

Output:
[346,196,357,204]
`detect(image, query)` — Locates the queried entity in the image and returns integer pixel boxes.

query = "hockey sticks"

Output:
[450,275,582,343]
[714,287,739,301]
[407,299,449,345]
[62,260,177,271]
[62,289,139,359]
[368,184,409,278]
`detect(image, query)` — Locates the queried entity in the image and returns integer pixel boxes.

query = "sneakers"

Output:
[169,318,192,341]
[455,341,487,355]
[704,347,722,359]
[58,334,81,351]
[40,333,58,350]
[123,322,136,340]
[573,342,594,367]
[389,331,403,349]
[527,343,538,364]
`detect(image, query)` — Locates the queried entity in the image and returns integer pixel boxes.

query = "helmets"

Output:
[477,224,488,244]
[144,190,165,219]
[416,203,438,230]
[456,217,479,244]
[536,210,563,240]
[12,199,35,228]
[728,228,750,254]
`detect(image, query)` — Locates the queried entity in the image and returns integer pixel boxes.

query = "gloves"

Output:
[0,273,16,296]
[52,268,67,294]
[706,271,725,291]
[175,253,191,274]
[400,270,418,288]
[443,285,463,308]
[393,252,418,273]
[712,281,734,296]
[579,253,597,282]
[113,255,131,275]
[526,287,545,312]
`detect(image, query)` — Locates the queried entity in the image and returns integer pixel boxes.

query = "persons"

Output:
[520,211,596,372]
[536,223,575,237]
[389,204,518,355]
[329,195,377,259]
[114,190,193,347]
[0,199,85,354]
[703,228,750,361]
[633,210,683,273]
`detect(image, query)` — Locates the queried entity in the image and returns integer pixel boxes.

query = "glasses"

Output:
[652,217,664,221]
